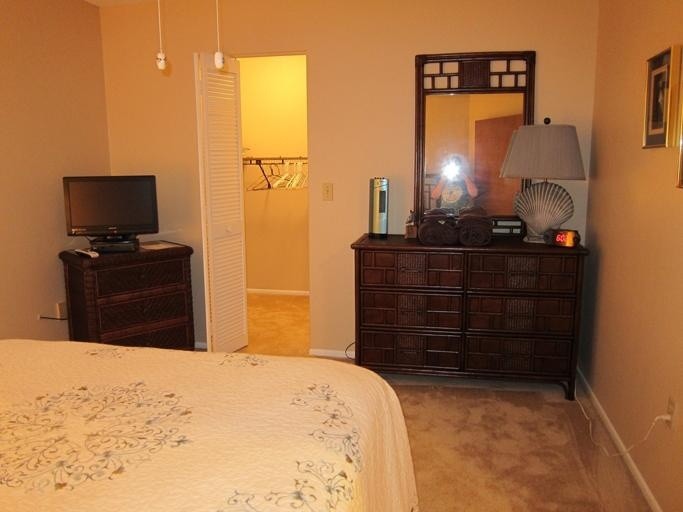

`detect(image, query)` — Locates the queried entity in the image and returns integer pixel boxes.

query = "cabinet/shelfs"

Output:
[351,232,463,381]
[56,240,195,349]
[463,234,591,401]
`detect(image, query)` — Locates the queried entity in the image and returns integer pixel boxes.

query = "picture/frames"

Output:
[640,45,673,148]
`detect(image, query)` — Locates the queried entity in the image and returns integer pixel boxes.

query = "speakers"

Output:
[373,177,389,240]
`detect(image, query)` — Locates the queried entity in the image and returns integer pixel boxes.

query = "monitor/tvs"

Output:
[63,176,158,246]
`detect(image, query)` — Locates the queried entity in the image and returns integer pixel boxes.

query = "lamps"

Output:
[502,116,588,244]
[211,2,224,72]
[151,2,166,73]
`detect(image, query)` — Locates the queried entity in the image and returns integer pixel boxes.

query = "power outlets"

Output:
[660,398,674,425]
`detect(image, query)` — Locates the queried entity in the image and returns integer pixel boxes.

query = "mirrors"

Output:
[416,92,526,215]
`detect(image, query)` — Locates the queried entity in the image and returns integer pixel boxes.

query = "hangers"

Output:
[250,163,308,192]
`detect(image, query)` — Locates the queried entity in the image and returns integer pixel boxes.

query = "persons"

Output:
[430,156,477,213]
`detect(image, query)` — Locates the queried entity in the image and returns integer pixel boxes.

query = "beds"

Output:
[0,337,420,512]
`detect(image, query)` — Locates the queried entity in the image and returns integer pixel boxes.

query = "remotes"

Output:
[74,249,99,258]
